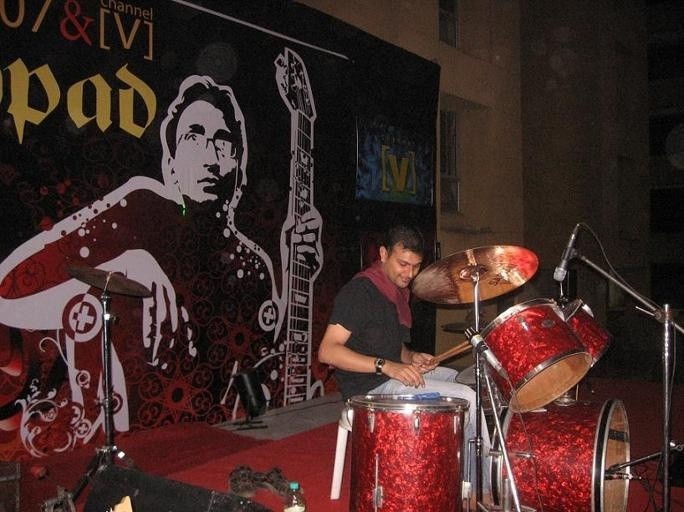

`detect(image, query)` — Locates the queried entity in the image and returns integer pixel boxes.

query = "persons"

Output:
[319,216,500,510]
[1,73,322,442]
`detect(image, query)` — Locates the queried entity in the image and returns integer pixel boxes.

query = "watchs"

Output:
[374,357,385,377]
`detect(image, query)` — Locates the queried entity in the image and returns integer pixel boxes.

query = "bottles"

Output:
[282,482,306,512]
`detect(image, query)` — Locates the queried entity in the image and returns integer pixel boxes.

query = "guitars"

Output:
[249,46,334,403]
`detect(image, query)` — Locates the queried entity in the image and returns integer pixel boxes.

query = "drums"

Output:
[474,297,591,414]
[564,296,612,370]
[350,395,470,512]
[489,397,631,512]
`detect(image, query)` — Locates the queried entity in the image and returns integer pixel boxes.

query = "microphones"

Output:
[464,327,509,381]
[553,224,580,282]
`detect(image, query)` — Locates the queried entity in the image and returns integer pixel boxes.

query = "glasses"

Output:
[176,132,239,160]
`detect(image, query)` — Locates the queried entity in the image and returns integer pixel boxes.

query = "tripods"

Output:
[61,292,142,510]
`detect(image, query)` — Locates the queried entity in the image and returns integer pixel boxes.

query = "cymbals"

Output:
[410,244,539,305]
[64,263,152,296]
